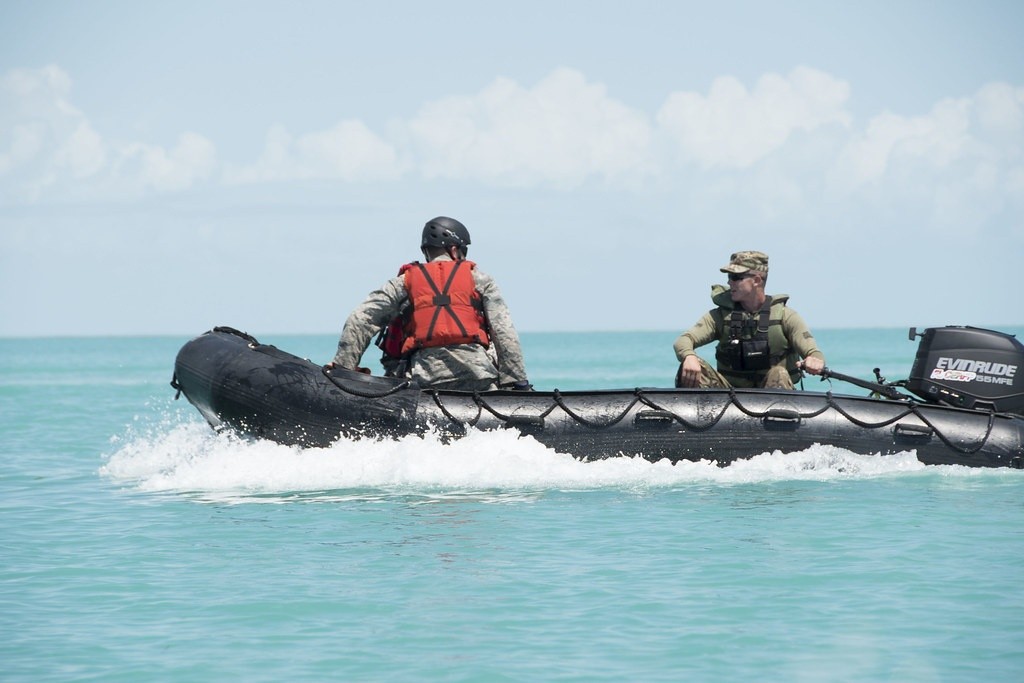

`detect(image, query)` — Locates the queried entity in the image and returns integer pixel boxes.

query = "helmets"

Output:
[421,216,471,257]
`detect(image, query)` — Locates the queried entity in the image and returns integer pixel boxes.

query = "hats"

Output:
[720,250,769,274]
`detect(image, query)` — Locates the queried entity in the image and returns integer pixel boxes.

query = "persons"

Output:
[673,251,825,390]
[327,215,535,392]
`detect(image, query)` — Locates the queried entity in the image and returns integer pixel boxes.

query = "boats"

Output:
[169,327,1024,469]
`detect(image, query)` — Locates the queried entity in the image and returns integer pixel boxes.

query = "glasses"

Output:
[728,274,755,282]
